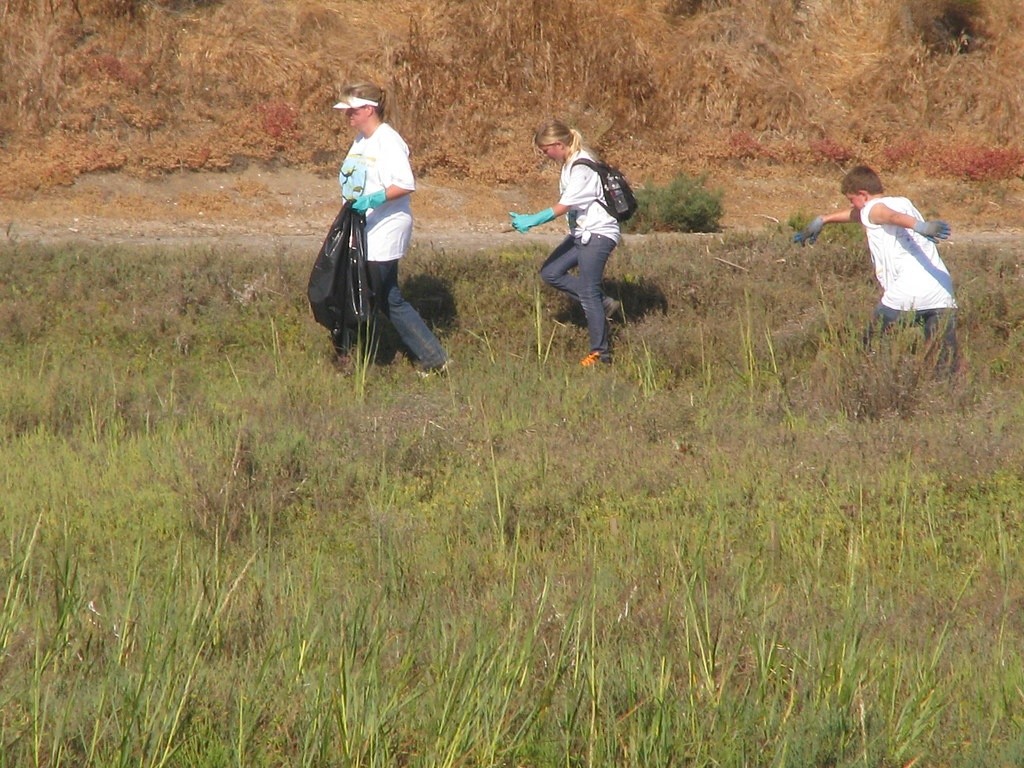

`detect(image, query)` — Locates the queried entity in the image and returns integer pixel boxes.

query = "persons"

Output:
[508,118,621,366]
[332,80,455,378]
[793,166,959,379]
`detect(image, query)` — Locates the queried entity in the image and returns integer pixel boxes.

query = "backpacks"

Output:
[570,159,636,222]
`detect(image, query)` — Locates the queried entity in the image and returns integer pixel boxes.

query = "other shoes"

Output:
[419,359,452,379]
[604,300,619,318]
[579,353,610,368]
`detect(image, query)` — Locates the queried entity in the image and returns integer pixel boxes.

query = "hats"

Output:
[333,95,379,109]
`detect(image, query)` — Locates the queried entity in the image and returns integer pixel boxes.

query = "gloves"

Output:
[508,207,556,234]
[351,191,388,216]
[793,217,824,248]
[914,220,952,244]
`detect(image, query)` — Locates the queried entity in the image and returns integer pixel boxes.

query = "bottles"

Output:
[608,177,627,213]
